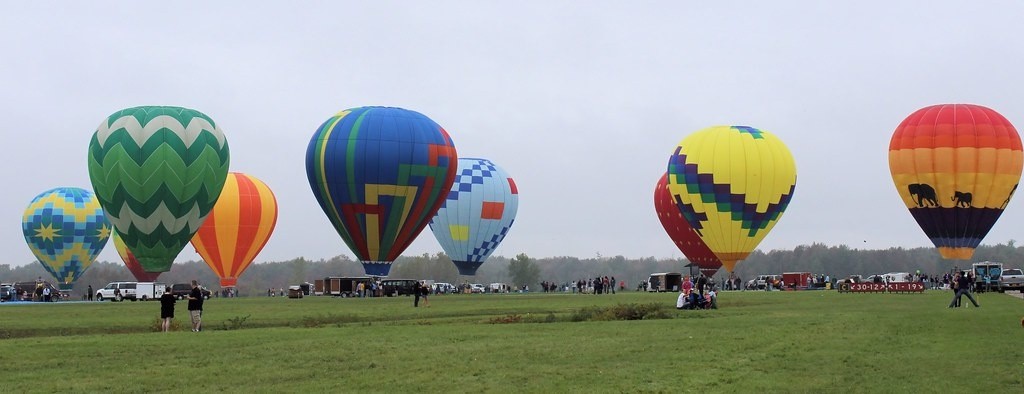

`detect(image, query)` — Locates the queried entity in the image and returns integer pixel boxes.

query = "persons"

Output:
[744,280,758,291]
[542,281,557,292]
[620,280,624,289]
[215,288,239,298]
[267,287,276,296]
[793,277,797,291]
[637,280,647,292]
[187,280,205,332]
[6,276,51,302]
[437,280,472,294]
[975,272,983,293]
[562,276,616,295]
[160,287,176,332]
[429,285,433,294]
[765,271,980,308]
[676,274,718,310]
[394,283,398,298]
[356,279,384,298]
[484,284,529,295]
[720,277,742,291]
[114,287,122,301]
[413,281,430,308]
[281,287,283,296]
[984,273,991,292]
[88,285,93,301]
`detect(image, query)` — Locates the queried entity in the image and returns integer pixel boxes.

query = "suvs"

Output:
[0,281,63,303]
[647,272,661,293]
[96,282,136,302]
[997,269,1024,293]
[167,283,212,300]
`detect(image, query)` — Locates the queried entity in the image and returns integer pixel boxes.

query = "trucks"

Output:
[299,277,381,297]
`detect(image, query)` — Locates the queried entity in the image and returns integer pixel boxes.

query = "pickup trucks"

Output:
[837,274,885,285]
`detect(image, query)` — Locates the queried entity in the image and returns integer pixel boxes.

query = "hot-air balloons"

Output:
[88,106,230,282]
[22,187,112,300]
[190,172,278,297]
[667,125,798,291]
[428,157,519,294]
[889,105,1024,278]
[654,170,723,288]
[305,106,458,297]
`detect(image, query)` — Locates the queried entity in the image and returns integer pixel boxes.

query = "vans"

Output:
[430,282,513,294]
[380,279,428,297]
[744,275,783,289]
